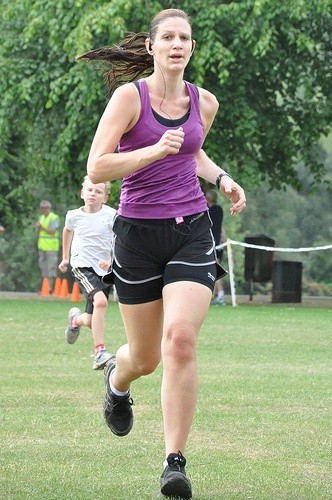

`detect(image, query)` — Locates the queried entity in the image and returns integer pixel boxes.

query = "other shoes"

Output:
[210,296,226,306]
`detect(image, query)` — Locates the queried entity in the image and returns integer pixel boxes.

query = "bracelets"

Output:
[215,173,232,190]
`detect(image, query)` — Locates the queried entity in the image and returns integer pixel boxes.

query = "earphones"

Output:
[149,42,152,50]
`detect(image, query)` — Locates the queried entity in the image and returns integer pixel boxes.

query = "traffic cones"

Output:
[38,277,51,297]
[51,277,69,300]
[69,281,83,302]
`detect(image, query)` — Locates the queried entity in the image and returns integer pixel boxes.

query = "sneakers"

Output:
[102,358,133,436]
[160,450,192,499]
[66,308,81,344]
[92,350,116,370]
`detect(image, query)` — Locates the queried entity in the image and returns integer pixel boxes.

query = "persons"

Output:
[203,190,225,304]
[87,10,246,499]
[35,199,61,293]
[58,173,118,370]
